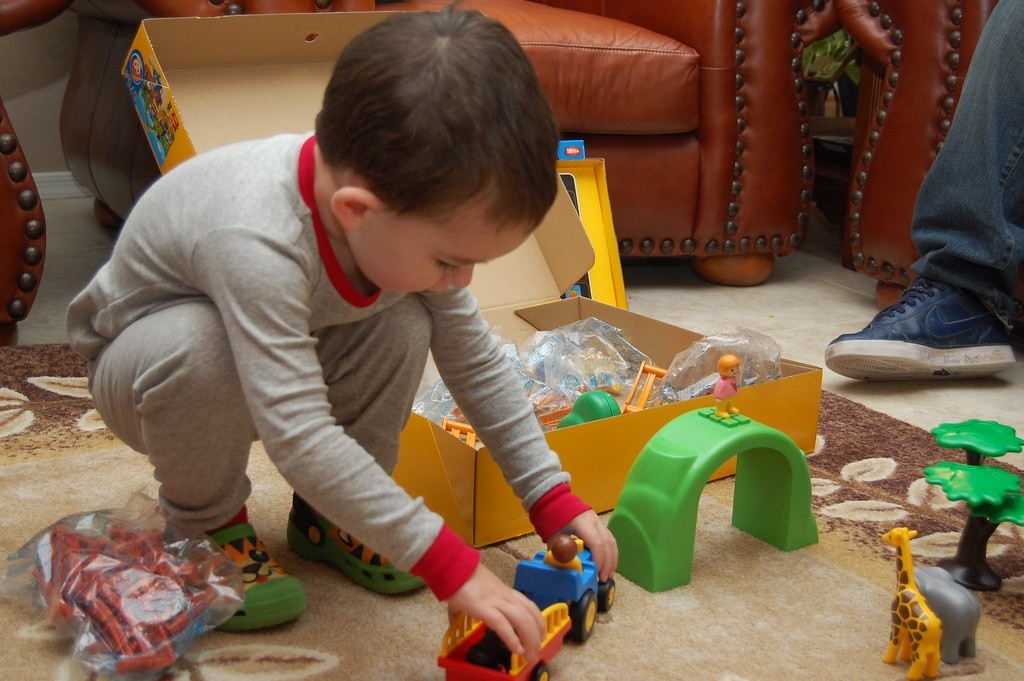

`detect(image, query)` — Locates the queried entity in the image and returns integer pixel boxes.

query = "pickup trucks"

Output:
[435,531,619,681]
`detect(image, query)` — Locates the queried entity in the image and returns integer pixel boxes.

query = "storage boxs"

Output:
[122,10,824,551]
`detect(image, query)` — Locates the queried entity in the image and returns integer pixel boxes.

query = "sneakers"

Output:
[825,273,1015,380]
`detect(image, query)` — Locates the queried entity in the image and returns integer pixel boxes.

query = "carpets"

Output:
[1,342,1024,676]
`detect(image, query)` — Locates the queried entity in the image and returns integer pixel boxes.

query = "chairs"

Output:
[56,0,812,291]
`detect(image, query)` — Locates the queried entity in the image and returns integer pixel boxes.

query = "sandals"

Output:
[287,490,426,594]
[207,522,308,631]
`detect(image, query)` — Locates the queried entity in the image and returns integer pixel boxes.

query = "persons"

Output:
[62,6,622,664]
[813,0,1024,379]
